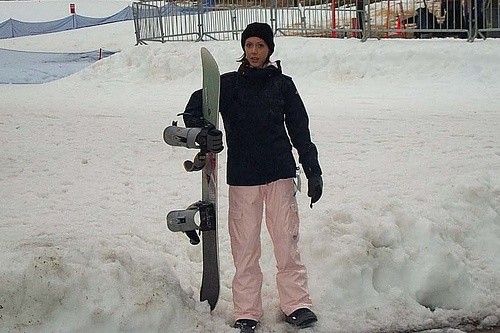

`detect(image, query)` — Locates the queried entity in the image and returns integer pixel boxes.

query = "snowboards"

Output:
[163,46,220,311]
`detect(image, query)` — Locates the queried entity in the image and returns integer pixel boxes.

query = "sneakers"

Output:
[285,305,317,325]
[231,319,256,333]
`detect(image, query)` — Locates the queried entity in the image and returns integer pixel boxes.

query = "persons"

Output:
[183,22,324,333]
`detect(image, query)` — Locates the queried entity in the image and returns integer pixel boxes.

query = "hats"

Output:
[241,23,274,52]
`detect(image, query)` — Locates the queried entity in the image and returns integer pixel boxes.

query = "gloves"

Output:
[197,118,223,154]
[303,161,322,209]
